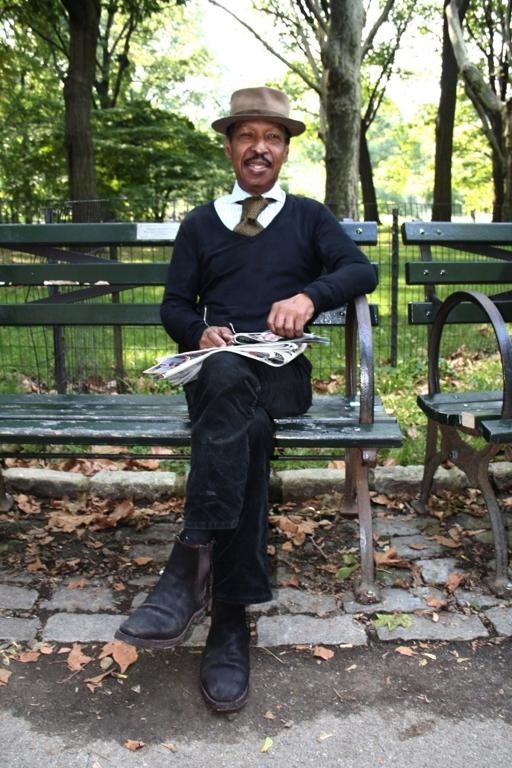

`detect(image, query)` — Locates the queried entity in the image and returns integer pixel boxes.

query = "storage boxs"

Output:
[0,219,405,606]
[400,217,511,596]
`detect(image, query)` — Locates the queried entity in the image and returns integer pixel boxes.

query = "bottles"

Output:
[210,86,307,137]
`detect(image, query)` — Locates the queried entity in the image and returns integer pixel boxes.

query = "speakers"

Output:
[198,599,251,714]
[114,533,216,652]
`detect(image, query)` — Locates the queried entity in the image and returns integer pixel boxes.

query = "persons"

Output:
[114,86,378,713]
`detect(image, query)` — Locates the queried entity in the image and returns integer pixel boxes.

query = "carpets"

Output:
[232,194,270,237]
